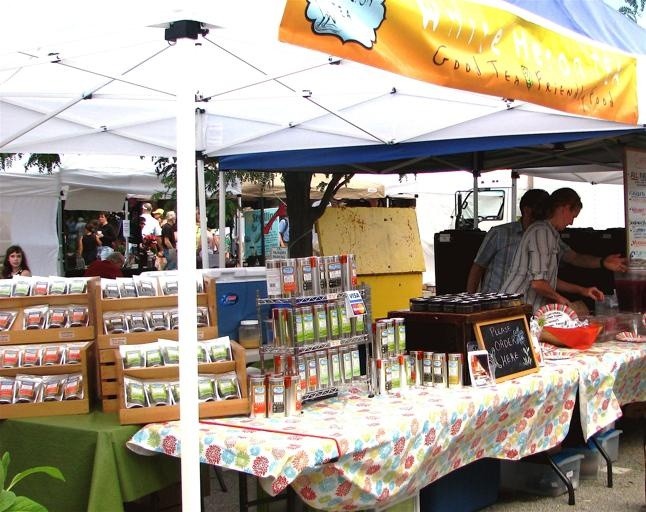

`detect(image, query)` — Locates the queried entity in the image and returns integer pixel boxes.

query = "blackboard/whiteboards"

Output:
[473,315,539,384]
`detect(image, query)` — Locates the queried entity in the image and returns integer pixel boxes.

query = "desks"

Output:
[125,359,579,509]
[3,411,181,511]
[540,339,645,490]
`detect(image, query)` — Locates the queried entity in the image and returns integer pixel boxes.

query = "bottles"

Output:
[410,350,463,388]
[374,318,407,392]
[239,320,261,349]
[273,344,360,390]
[272,299,366,347]
[248,373,302,419]
[266,253,360,298]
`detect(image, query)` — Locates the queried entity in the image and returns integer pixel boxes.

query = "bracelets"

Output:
[600,256,605,269]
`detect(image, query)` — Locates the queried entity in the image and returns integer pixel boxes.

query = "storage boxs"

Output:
[599,427,624,463]
[561,435,606,480]
[381,492,420,511]
[539,450,585,498]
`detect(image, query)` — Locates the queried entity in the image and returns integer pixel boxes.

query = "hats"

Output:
[152,208,164,214]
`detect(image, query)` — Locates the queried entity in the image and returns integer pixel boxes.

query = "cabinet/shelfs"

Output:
[255,282,374,406]
[0,275,251,425]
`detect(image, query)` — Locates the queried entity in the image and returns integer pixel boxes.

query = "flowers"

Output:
[141,234,160,255]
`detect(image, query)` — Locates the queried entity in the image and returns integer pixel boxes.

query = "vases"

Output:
[146,249,154,268]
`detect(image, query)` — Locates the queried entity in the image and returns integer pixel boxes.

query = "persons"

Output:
[277,217,289,248]
[65,202,235,280]
[498,188,605,315]
[466,189,628,295]
[0,244,31,279]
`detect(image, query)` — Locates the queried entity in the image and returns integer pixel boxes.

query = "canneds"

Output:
[409,291,524,314]
[250,254,462,419]
